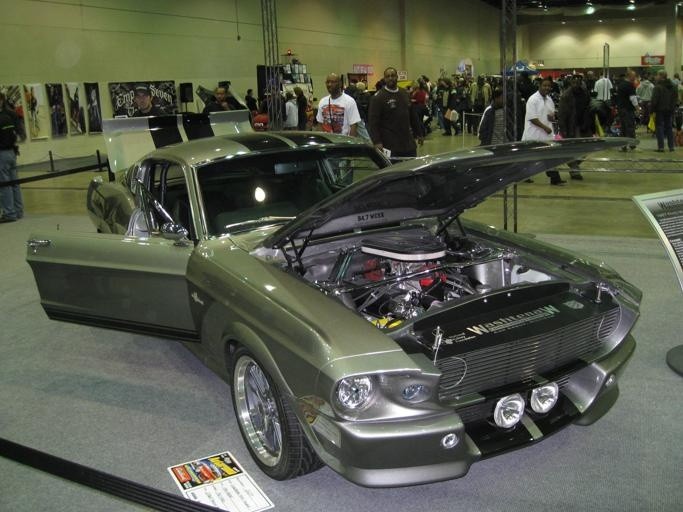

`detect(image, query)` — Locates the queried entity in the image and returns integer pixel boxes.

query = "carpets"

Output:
[1,210,683,512]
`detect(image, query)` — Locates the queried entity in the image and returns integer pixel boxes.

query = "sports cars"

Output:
[24,107,647,493]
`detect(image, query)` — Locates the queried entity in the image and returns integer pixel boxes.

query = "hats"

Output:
[132,83,152,96]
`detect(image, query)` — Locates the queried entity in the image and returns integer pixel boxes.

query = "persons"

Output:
[131,82,167,117]
[0,93,24,223]
[315,67,682,184]
[283,86,309,129]
[204,80,257,115]
[71,87,80,129]
[23,85,41,138]
